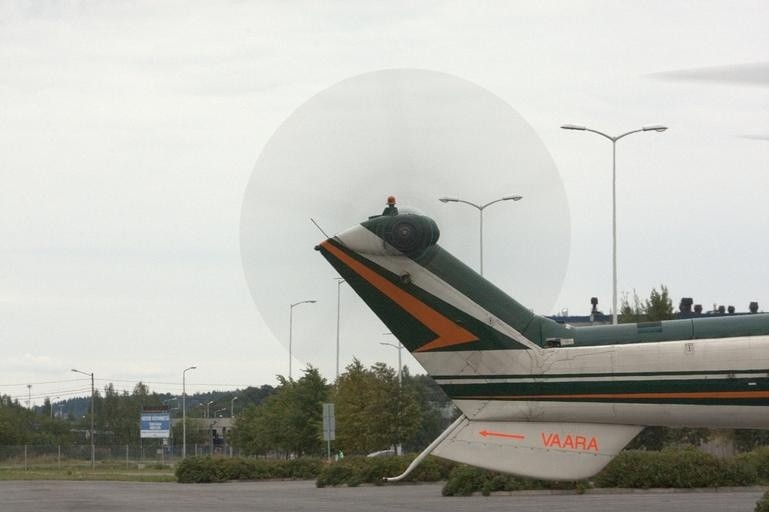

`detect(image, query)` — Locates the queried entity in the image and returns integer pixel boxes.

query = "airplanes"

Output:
[312,197,769,484]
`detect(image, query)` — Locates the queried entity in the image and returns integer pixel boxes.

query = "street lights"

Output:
[439,195,521,276]
[288,300,316,377]
[183,366,197,459]
[561,124,668,323]
[381,342,405,426]
[72,369,95,467]
